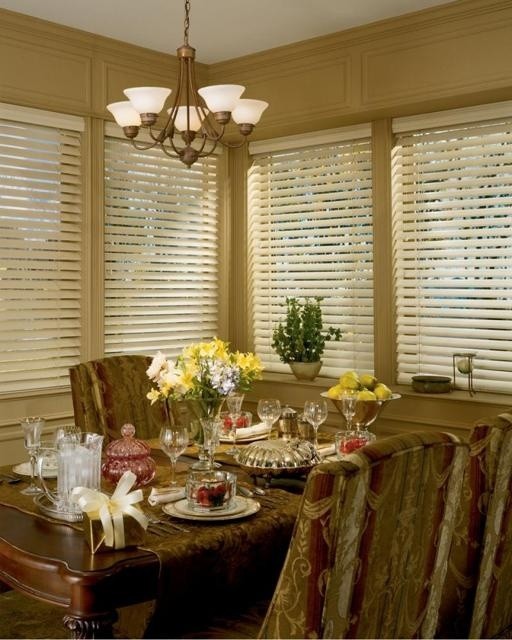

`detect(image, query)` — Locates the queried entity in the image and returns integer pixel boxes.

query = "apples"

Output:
[327,371,393,401]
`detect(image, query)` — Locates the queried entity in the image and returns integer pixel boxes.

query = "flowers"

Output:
[147,336,265,422]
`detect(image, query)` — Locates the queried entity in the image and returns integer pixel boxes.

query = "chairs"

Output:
[69,353,174,449]
[169,431,471,638]
[443,411,512,639]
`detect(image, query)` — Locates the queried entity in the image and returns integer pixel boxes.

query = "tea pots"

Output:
[40,431,107,509]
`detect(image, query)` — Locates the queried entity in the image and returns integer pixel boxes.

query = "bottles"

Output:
[297,412,314,442]
[277,405,298,438]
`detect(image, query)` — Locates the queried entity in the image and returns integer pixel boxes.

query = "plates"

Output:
[33,492,85,523]
[10,462,61,479]
[235,456,321,489]
[220,433,268,442]
[161,495,263,523]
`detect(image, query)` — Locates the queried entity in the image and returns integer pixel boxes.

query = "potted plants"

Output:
[270,295,345,381]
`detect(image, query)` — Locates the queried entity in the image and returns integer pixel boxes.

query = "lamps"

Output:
[105,0,271,169]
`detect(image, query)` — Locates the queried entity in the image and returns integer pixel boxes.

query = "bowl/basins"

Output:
[218,410,253,436]
[410,375,454,394]
[335,432,368,461]
[101,423,156,491]
[187,470,237,513]
[320,392,401,444]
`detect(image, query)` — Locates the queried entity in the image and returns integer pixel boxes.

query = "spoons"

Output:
[0,473,20,484]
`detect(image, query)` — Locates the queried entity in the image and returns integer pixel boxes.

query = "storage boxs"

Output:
[82,502,147,555]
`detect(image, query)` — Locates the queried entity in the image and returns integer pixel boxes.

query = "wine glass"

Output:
[198,418,222,475]
[224,394,246,456]
[256,397,281,440]
[303,397,329,453]
[19,416,82,499]
[337,391,360,432]
[158,426,188,486]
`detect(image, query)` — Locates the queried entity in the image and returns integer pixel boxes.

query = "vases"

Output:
[182,401,222,471]
